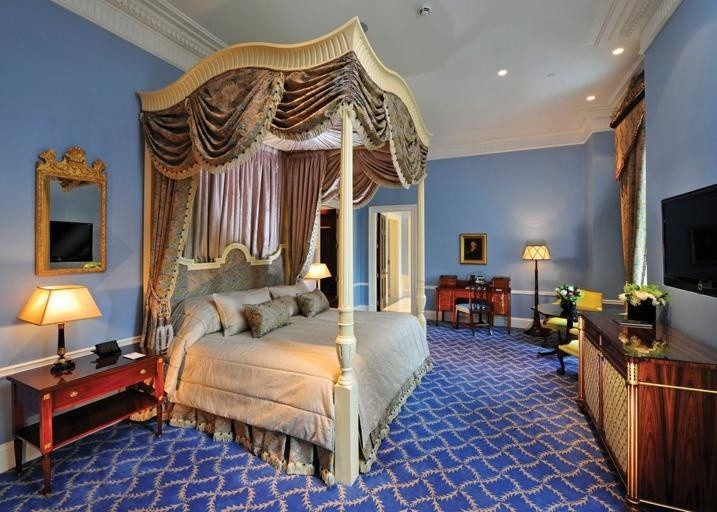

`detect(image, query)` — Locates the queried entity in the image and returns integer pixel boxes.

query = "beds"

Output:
[134,14,433,487]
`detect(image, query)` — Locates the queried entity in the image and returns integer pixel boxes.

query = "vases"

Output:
[560,299,576,312]
[626,299,656,323]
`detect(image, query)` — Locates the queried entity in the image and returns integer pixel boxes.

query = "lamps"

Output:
[304,263,333,291]
[18,285,102,366]
[521,245,552,338]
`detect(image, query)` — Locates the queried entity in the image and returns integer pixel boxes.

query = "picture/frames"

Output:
[460,233,487,264]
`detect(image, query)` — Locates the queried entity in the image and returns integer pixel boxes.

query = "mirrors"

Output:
[34,146,107,275]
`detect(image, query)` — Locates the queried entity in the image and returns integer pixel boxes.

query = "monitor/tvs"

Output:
[660,184,717,298]
[49,220,93,262]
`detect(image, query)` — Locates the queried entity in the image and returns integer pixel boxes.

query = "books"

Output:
[614,318,653,327]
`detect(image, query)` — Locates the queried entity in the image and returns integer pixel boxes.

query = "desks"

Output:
[531,303,597,361]
[435,275,511,334]
[7,344,166,499]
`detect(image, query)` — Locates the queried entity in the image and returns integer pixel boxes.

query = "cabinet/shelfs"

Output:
[577,310,717,512]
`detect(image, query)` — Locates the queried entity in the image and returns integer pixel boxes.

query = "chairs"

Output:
[453,280,494,335]
[546,290,603,345]
[557,339,581,375]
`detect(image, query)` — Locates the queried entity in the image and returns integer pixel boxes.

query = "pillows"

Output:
[212,281,329,338]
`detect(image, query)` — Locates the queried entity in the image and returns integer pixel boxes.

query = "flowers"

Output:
[618,332,672,359]
[555,282,582,302]
[623,280,670,307]
[84,260,102,271]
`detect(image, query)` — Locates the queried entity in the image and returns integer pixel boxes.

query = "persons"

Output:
[466,241,482,261]
